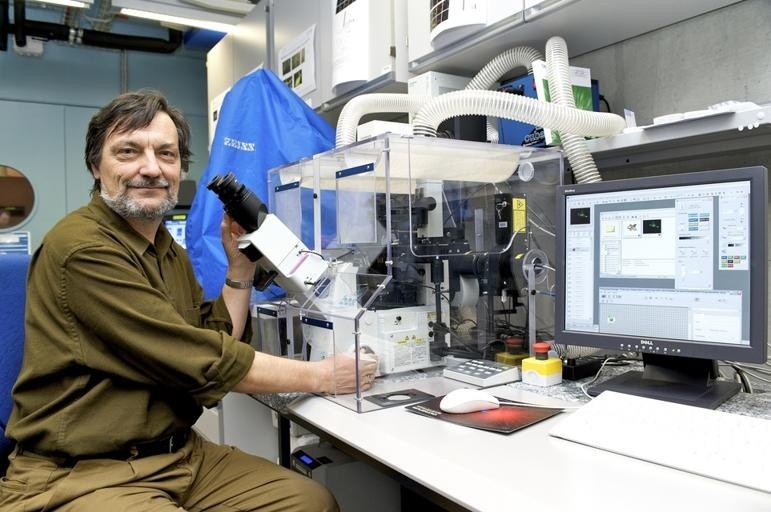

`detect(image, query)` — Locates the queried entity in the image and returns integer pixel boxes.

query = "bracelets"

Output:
[225,276,255,288]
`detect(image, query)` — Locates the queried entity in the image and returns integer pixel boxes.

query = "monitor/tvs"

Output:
[554,166,768,411]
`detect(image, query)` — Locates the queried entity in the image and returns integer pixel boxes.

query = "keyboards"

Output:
[549,388,771,495]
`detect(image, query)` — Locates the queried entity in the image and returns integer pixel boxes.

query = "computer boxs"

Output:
[291,442,402,512]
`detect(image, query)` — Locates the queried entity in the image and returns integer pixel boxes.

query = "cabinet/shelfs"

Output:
[206,0,408,155]
[408,0,747,84]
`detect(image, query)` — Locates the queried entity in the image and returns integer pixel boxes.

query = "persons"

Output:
[0,87,378,512]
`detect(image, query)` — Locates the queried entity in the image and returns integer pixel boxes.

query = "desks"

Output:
[248,357,770,511]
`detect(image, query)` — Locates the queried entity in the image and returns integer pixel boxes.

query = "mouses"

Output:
[439,388,499,413]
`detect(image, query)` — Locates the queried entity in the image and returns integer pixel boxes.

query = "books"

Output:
[531,57,594,146]
